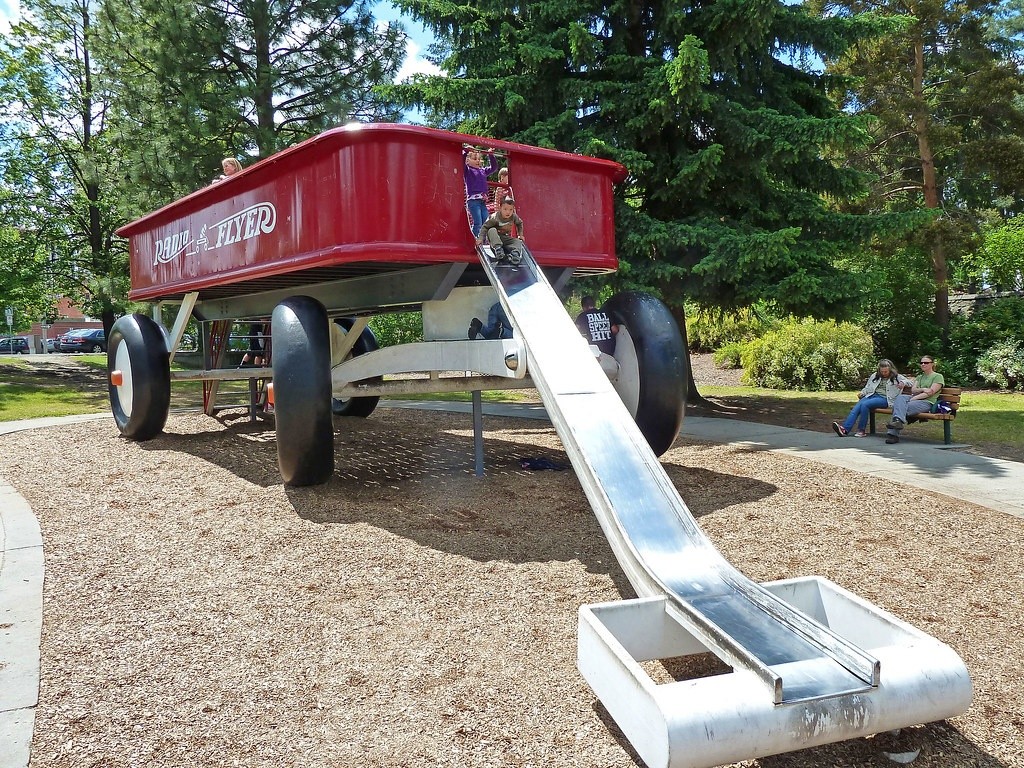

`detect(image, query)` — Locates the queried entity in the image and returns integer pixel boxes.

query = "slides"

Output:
[472,237,884,711]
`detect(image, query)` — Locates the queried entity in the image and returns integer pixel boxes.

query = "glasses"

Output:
[919,362,933,364]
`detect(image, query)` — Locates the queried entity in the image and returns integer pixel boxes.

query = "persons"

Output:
[885,355,945,443]
[476,197,526,264]
[237,319,264,369]
[494,168,508,211]
[573,295,618,355]
[463,146,498,236]
[832,358,913,436]
[211,158,242,184]
[468,302,513,339]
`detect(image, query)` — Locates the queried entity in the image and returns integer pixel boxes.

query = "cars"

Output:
[60,329,107,354]
[0,329,81,354]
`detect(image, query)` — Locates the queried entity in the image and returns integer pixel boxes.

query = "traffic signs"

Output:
[40,324,51,328]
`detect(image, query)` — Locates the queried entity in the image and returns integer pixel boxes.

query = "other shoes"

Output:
[506,253,520,264]
[491,321,504,339]
[493,246,505,260]
[885,435,899,444]
[237,365,244,369]
[854,432,867,437]
[832,421,848,437]
[885,420,904,429]
[468,317,483,340]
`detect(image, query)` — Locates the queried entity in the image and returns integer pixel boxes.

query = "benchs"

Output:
[870,388,961,443]
